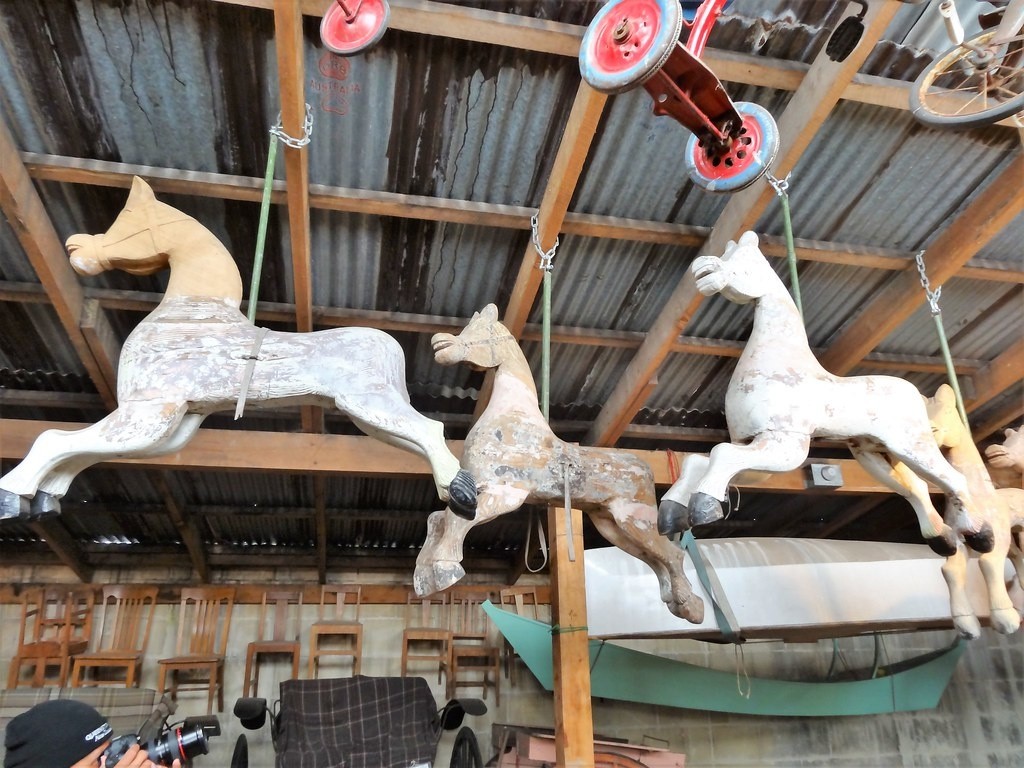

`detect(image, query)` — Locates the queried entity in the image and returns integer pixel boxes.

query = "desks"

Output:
[7,587,95,688]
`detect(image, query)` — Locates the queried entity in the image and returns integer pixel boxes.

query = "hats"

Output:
[4,699,113,768]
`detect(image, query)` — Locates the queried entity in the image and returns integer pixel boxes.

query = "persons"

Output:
[4,702,186,768]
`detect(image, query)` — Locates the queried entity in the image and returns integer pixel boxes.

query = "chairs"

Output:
[402,591,453,699]
[499,586,539,687]
[450,591,500,707]
[158,587,235,715]
[308,584,363,679]
[72,584,160,688]
[243,591,302,698]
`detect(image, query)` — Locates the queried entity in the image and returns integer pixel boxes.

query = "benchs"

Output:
[0,689,179,768]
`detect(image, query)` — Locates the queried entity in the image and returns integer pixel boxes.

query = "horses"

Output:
[0,175,1024,640]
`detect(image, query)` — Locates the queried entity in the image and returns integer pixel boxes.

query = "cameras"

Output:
[102,723,212,768]
